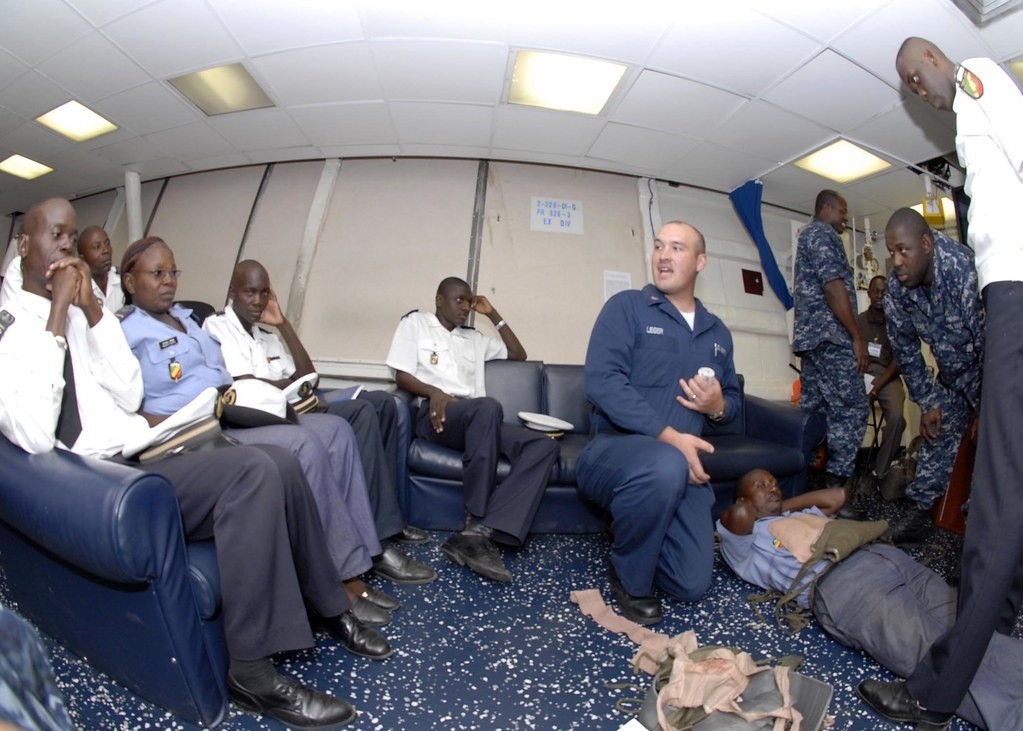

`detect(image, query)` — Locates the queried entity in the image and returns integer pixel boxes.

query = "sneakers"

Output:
[886,511,930,547]
[824,474,868,520]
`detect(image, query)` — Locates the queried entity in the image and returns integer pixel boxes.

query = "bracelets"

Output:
[496,319,506,331]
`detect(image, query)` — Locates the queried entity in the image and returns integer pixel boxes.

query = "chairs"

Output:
[863,380,904,475]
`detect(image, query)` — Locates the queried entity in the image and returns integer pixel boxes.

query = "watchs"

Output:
[709,403,728,422]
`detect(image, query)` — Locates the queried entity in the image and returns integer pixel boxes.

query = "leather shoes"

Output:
[349,596,391,627]
[226,668,356,730]
[370,542,438,585]
[391,526,431,544]
[609,564,661,624]
[856,679,953,731]
[333,613,392,660]
[465,509,471,528]
[441,533,512,581]
[358,584,400,611]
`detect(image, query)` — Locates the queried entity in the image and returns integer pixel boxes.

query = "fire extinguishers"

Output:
[789,363,824,469]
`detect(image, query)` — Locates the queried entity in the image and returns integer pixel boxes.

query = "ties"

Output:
[55,334,82,449]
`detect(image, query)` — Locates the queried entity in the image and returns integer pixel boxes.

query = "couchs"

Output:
[0,430,232,731]
[394,361,822,529]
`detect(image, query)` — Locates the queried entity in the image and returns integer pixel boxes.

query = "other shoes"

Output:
[860,470,878,495]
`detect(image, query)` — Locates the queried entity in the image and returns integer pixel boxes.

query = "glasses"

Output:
[132,270,182,279]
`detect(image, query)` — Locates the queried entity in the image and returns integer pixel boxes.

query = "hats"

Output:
[222,379,301,427]
[122,387,244,466]
[283,372,330,415]
[517,412,575,440]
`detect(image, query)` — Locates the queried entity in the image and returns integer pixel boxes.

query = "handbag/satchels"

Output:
[749,519,888,634]
[638,646,833,731]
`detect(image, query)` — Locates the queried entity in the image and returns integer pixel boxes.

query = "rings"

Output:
[688,395,696,401]
[432,412,437,417]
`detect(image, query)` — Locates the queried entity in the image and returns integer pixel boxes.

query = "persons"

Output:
[856,36,1023,731]
[791,190,871,520]
[573,221,742,625]
[385,278,562,583]
[881,207,984,547]
[856,275,906,498]
[0,198,438,731]
[716,468,1023,731]
